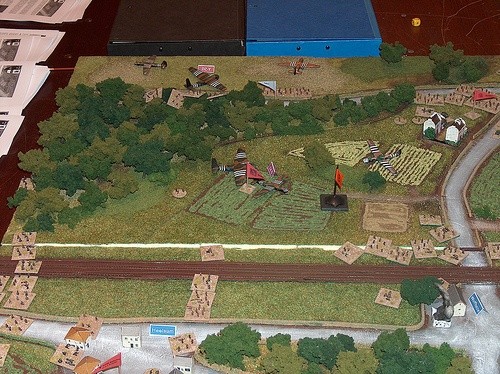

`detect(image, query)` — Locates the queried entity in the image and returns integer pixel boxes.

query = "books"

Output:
[0,0,92,158]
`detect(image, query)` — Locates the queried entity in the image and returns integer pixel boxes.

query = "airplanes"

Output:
[212,148,256,186]
[134,55,167,75]
[279,58,320,75]
[184,67,227,91]
